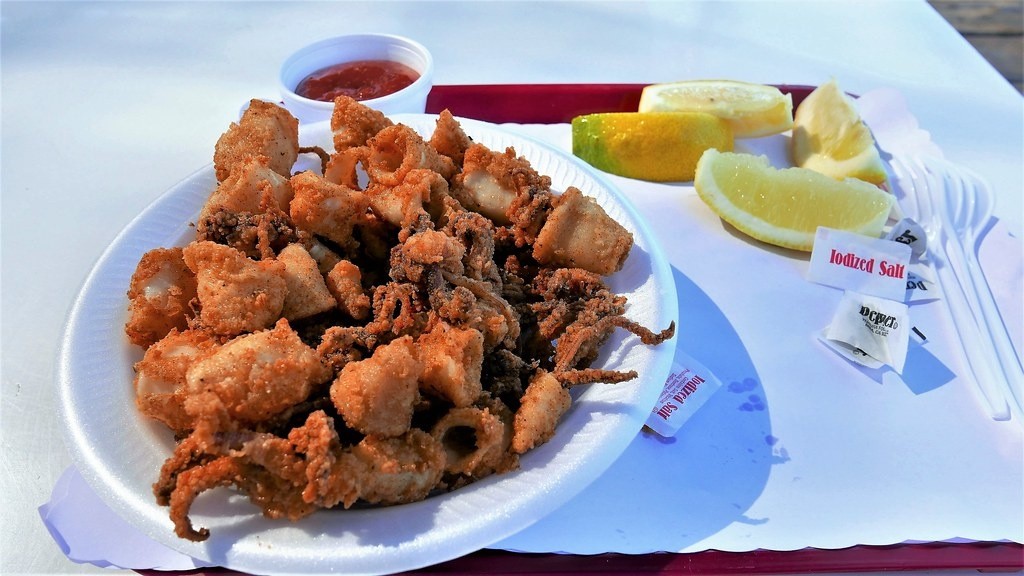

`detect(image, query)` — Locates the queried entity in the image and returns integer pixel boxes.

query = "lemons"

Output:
[571,80,897,253]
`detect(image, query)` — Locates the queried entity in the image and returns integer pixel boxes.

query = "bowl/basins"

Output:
[277,32,435,124]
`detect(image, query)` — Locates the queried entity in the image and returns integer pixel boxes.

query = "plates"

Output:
[57,113,678,576]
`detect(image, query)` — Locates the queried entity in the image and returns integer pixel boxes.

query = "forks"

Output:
[919,153,1024,411]
[880,154,1010,421]
